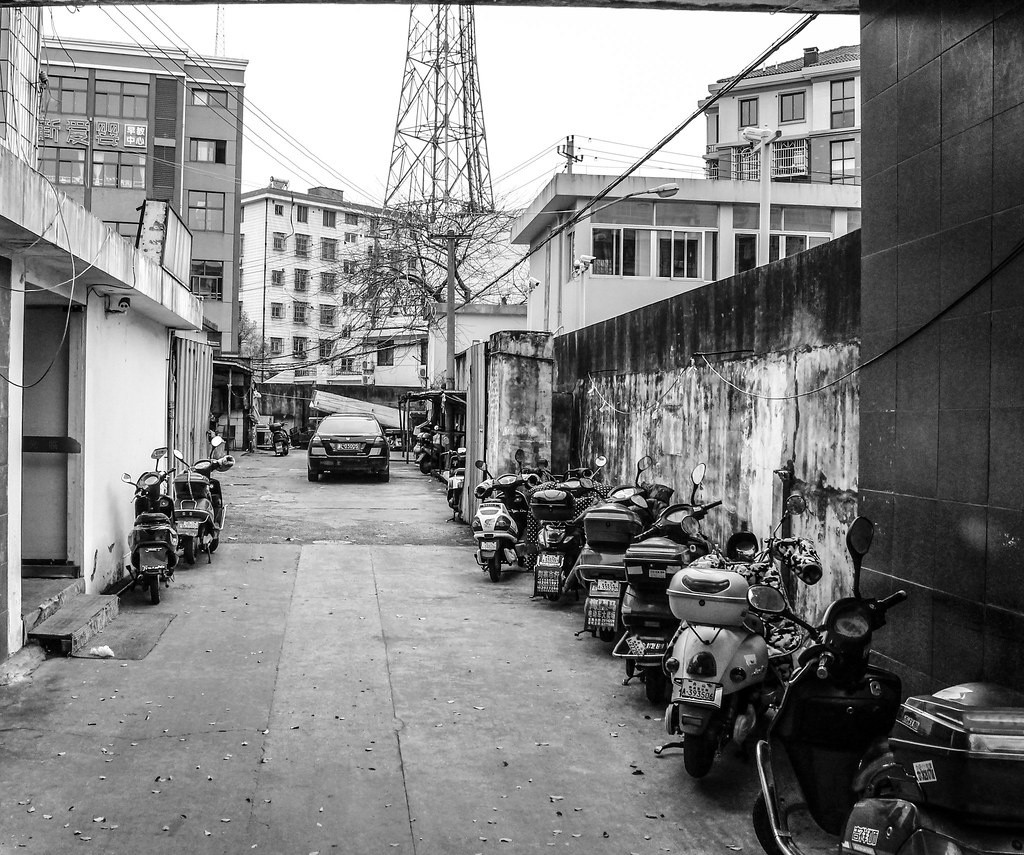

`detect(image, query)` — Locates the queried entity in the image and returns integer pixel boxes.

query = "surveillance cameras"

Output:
[573,255,596,268]
[118,297,131,311]
[744,127,763,141]
[528,277,541,286]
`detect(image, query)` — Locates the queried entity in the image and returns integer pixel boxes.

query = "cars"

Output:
[307,413,391,482]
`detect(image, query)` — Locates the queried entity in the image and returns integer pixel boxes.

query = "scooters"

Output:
[528,451,612,602]
[611,462,723,707]
[169,436,234,566]
[409,416,467,521]
[742,513,1023,855]
[470,446,542,582]
[652,490,823,778]
[120,447,177,605]
[571,454,672,639]
[267,414,291,456]
